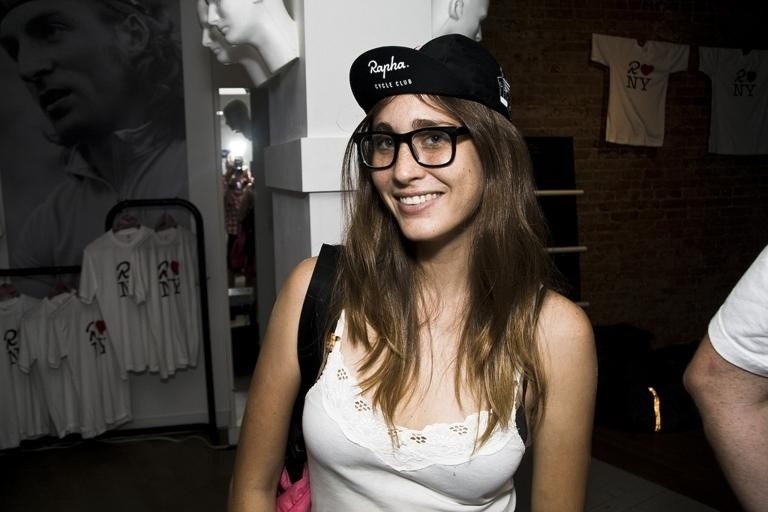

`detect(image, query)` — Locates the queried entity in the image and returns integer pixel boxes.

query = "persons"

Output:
[204,1,302,77]
[681,239,767,512]
[196,0,288,89]
[225,30,600,512]
[0,1,191,301]
[222,156,256,290]
[221,98,252,143]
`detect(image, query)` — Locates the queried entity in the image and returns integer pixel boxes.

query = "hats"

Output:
[349,33,511,120]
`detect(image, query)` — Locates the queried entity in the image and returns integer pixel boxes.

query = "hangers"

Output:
[5,200,178,301]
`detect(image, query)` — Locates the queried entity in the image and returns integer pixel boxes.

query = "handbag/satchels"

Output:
[276,243,350,512]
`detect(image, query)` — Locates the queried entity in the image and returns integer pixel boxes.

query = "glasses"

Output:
[353,126,470,170]
